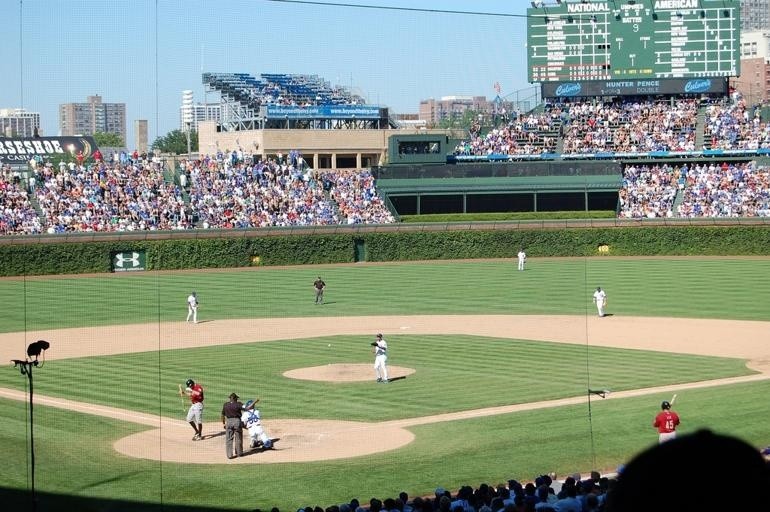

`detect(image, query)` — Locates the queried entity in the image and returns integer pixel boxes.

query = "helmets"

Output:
[186,379,194,388]
[246,400,254,409]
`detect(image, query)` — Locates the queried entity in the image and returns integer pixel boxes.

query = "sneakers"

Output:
[193,433,202,441]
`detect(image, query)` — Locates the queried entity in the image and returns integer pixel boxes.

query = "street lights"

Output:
[182,88,194,161]
[11,341,49,512]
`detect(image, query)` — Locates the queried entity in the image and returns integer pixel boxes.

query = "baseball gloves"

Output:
[371,342,377,346]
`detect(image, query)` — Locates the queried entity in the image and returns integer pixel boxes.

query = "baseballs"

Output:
[328,344,331,347]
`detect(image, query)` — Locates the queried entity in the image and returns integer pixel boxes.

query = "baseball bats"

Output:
[179,384,185,412]
[669,395,677,406]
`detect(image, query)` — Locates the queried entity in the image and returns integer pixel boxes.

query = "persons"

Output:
[313,276,326,304]
[178,379,206,443]
[592,286,607,317]
[517,249,526,271]
[186,291,200,324]
[0,148,396,236]
[653,400,680,443]
[220,392,261,458]
[596,426,770,510]
[240,398,274,449]
[250,442,770,512]
[617,161,770,221]
[452,93,770,157]
[372,333,389,384]
[251,82,360,107]
